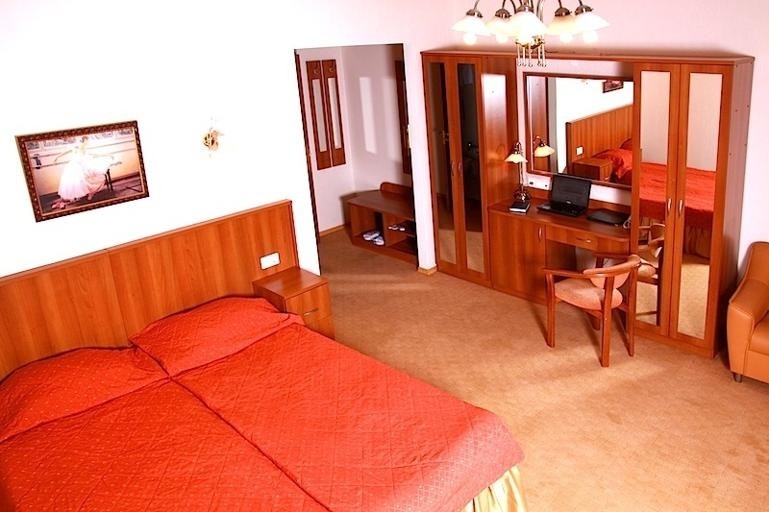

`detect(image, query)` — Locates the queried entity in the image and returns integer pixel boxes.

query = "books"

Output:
[509,200,530,212]
[587,208,630,226]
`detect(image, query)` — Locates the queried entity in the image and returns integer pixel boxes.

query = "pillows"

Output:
[594,138,632,178]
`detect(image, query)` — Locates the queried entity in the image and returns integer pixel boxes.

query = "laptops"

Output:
[537,174,591,216]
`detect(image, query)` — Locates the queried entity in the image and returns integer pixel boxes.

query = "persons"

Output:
[48,136,122,204]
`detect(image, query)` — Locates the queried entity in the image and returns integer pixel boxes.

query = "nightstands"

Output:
[251,266,335,341]
[570,158,613,182]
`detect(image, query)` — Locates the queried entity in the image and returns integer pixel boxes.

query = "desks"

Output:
[487,198,630,334]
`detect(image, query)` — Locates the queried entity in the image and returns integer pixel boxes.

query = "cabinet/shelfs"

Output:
[419,48,519,290]
[632,55,755,360]
[345,182,419,267]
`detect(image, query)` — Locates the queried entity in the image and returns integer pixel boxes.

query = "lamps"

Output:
[532,135,556,159]
[503,141,532,202]
[449,0,609,69]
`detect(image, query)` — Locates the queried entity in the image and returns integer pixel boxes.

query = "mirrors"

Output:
[424,60,486,276]
[522,71,635,192]
[638,69,724,340]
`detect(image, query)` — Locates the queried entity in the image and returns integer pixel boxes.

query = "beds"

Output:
[0,324,526,512]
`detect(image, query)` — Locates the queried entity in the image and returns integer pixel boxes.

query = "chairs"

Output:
[539,252,641,368]
[726,241,769,383]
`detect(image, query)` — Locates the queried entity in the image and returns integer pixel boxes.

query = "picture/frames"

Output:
[601,80,624,95]
[14,119,150,223]
